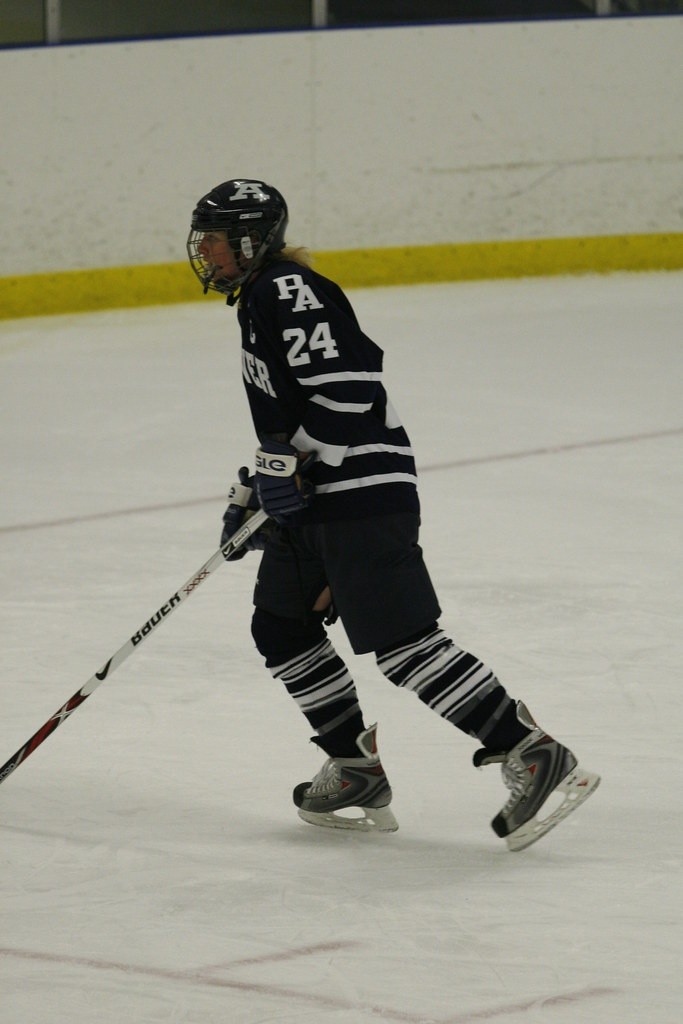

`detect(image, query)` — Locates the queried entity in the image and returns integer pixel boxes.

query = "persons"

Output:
[185,180,602,855]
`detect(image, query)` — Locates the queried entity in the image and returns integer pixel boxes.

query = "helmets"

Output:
[186,179,289,293]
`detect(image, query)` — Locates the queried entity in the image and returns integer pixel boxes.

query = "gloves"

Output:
[256,441,319,538]
[220,466,272,561]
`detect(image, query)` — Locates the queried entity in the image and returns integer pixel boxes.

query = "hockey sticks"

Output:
[0,449,322,783]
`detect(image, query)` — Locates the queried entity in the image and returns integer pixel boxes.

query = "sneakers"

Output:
[292,722,400,833]
[472,700,601,852]
